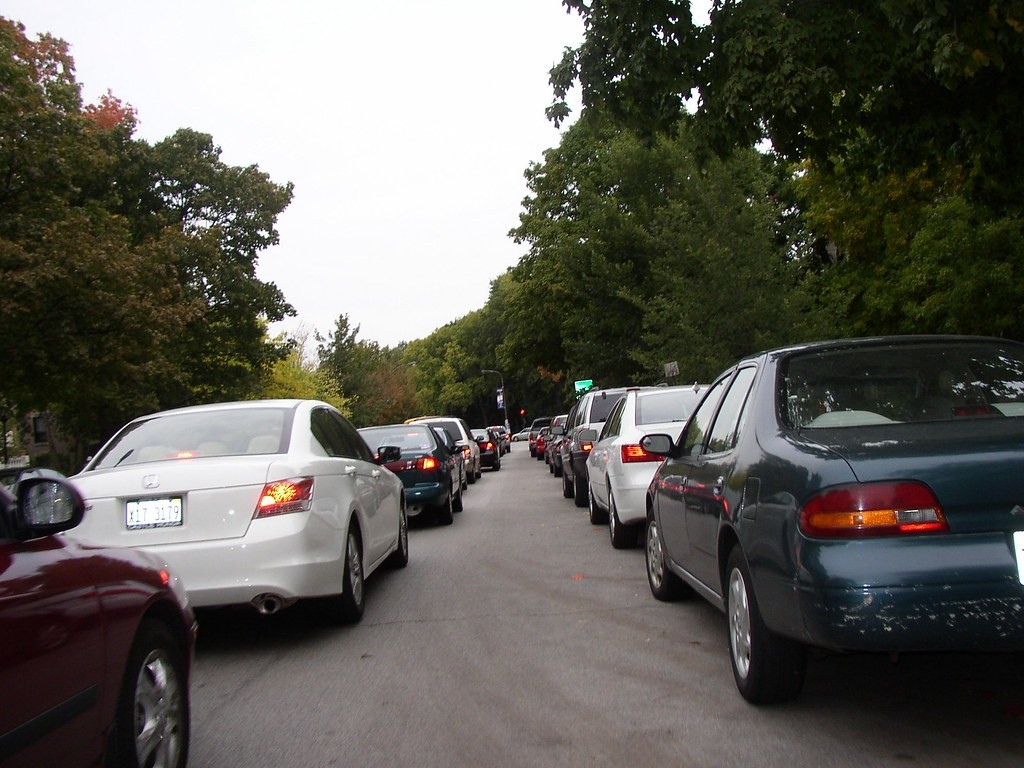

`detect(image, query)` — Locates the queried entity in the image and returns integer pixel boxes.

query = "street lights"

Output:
[481,370,509,430]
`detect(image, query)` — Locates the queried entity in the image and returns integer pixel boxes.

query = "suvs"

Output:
[549,382,670,508]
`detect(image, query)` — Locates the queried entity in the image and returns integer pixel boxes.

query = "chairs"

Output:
[794,374,845,428]
[135,446,178,463]
[247,435,280,453]
[842,372,898,423]
[923,359,989,424]
[197,442,230,455]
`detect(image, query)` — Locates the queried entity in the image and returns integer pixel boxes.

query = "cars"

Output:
[1,465,196,768]
[354,421,466,526]
[378,412,568,495]
[636,332,1024,706]
[51,399,411,625]
[577,380,723,549]
